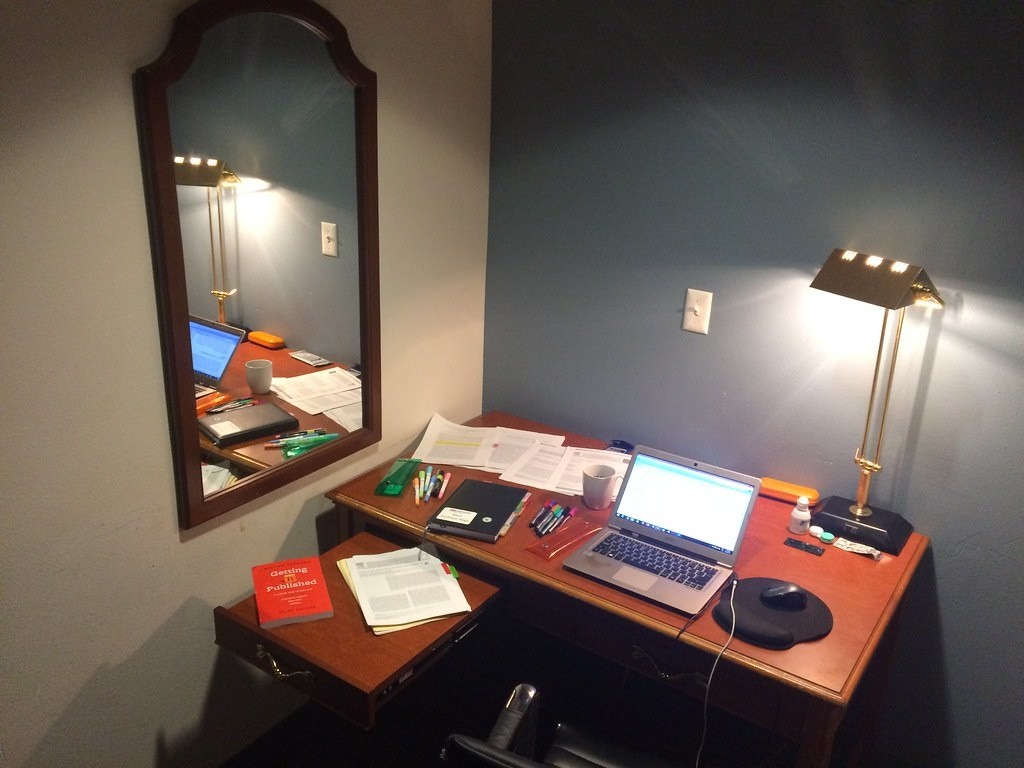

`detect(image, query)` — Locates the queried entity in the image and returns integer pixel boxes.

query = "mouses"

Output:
[762,584,809,609]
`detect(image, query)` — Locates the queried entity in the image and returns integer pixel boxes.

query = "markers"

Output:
[263,426,327,447]
[530,499,579,538]
[412,464,452,505]
[204,396,263,414]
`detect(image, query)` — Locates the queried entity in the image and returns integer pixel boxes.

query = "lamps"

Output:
[805,248,945,558]
[173,152,253,344]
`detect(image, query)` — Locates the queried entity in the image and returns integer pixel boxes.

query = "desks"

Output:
[214,411,931,767]
[196,341,360,471]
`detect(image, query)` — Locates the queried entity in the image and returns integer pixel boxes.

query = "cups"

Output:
[245,359,271,394]
[583,465,623,510]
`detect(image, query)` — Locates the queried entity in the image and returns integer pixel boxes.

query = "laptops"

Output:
[562,444,761,615]
[189,314,245,398]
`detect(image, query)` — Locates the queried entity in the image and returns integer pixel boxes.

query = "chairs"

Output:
[441,683,790,767]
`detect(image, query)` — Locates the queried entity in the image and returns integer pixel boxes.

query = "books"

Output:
[197,401,301,446]
[253,555,335,631]
[427,478,533,543]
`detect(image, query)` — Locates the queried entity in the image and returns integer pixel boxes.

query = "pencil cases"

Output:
[524,515,603,561]
[196,391,230,417]
[374,457,422,496]
[281,433,340,460]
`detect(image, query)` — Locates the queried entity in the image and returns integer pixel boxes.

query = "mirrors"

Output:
[125,0,386,530]
